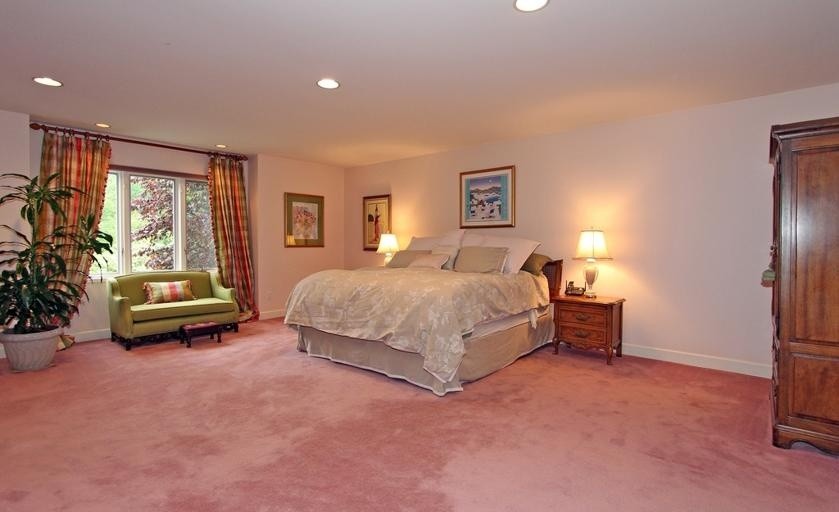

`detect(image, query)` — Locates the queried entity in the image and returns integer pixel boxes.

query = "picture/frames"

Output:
[459,165,515,228]
[284,192,325,248]
[363,194,392,250]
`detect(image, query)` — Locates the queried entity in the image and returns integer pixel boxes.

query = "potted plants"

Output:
[0,169,114,373]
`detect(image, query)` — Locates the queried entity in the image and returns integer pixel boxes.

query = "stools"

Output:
[181,323,222,347]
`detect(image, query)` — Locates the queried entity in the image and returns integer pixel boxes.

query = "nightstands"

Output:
[551,295,626,364]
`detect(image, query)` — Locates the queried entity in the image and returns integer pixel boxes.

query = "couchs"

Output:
[108,270,240,351]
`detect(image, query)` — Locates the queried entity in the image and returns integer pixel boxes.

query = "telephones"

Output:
[564,280,586,295]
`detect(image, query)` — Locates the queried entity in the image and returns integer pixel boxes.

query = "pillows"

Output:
[143,280,196,304]
[386,228,553,277]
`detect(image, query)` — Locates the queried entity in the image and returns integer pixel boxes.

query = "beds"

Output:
[283,258,563,398]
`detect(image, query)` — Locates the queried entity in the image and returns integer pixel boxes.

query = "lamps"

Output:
[376,234,400,266]
[572,230,613,299]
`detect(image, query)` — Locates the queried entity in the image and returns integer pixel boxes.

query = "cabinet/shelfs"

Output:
[761,114,839,455]
